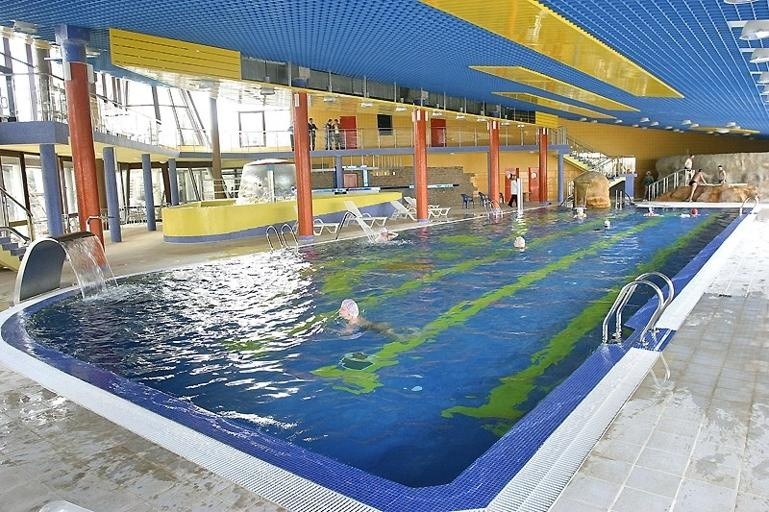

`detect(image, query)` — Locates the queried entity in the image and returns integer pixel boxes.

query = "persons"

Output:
[644,205,657,216]
[683,153,695,176]
[287,118,345,151]
[691,208,699,216]
[322,297,390,338]
[513,235,527,251]
[687,168,707,202]
[546,202,588,219]
[718,165,727,184]
[506,175,517,208]
[641,170,654,200]
[601,220,611,229]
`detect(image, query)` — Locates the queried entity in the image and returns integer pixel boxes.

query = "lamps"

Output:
[323,70,336,102]
[260,59,275,94]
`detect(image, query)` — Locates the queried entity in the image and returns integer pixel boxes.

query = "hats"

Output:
[692,209,698,214]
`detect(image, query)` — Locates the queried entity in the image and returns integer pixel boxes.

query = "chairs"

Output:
[290,197,451,236]
[460,192,504,208]
